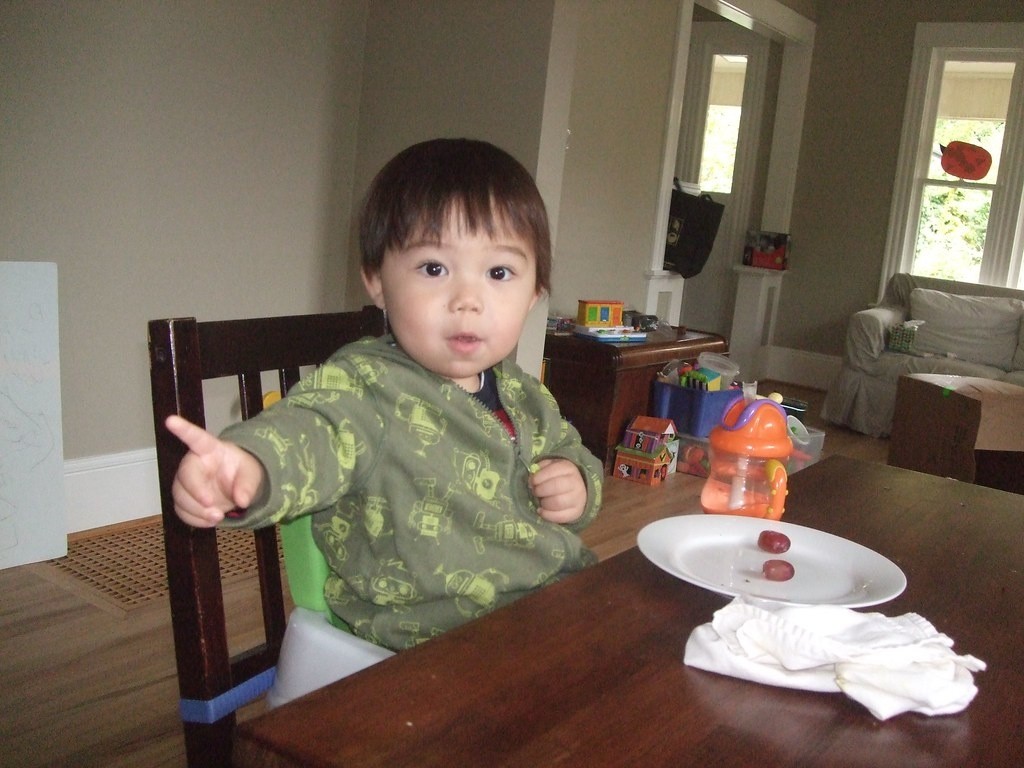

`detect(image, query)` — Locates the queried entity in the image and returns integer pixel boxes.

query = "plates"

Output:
[637,514,908,609]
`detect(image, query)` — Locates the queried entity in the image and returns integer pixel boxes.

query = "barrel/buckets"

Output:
[697,351,740,390]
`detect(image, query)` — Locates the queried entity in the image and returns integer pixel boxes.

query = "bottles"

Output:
[700,381,794,520]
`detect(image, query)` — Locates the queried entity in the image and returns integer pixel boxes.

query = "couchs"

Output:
[819,272,1024,437]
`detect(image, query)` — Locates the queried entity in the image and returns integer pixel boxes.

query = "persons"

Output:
[163,135,604,647]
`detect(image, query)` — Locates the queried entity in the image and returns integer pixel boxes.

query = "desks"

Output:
[231,451,1024,768]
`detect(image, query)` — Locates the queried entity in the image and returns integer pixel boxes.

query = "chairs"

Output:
[145,304,388,768]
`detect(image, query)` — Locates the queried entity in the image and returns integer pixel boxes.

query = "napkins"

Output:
[684,596,986,721]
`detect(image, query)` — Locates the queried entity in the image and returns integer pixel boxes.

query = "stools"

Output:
[888,373,1024,496]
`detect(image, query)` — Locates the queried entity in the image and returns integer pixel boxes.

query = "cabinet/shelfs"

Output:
[542,325,729,475]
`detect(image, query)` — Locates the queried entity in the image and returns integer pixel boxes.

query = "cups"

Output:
[787,415,811,447]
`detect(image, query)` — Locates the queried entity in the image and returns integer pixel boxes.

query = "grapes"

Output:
[758,530,791,554]
[763,560,795,581]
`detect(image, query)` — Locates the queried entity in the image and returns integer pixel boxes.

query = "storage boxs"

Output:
[676,427,827,482]
[654,379,743,436]
